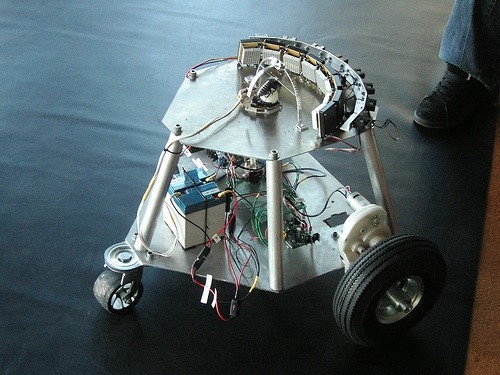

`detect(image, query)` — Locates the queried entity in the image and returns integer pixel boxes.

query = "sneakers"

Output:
[413,63,488,133]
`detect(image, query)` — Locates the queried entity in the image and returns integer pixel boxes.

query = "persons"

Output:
[413,0,500,129]
